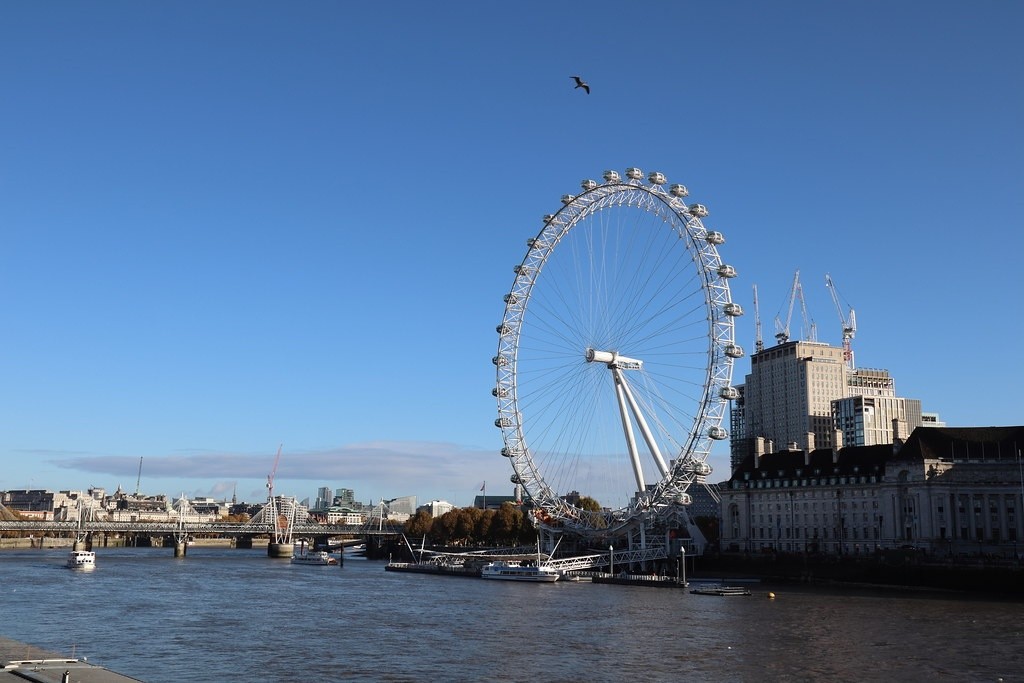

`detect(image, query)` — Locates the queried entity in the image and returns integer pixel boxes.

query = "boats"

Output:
[67,550,96,573]
[480,534,563,582]
[385,532,479,574]
[0,637,147,683]
[290,551,338,565]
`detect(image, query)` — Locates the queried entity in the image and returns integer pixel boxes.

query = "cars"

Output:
[901,545,919,552]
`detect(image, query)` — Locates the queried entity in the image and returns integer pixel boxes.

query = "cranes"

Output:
[751,283,764,353]
[824,271,857,365]
[265,443,283,497]
[773,269,818,344]
